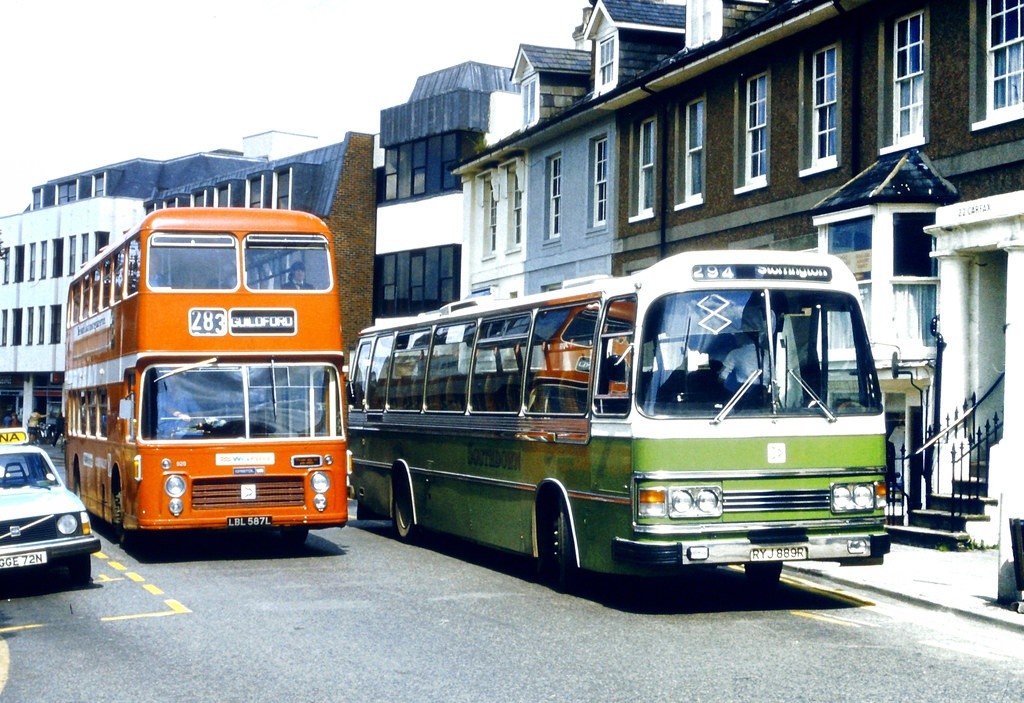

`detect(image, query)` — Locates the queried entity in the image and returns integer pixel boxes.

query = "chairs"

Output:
[368,374,585,415]
[598,354,625,394]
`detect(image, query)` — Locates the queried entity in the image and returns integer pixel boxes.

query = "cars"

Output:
[0,427,103,591]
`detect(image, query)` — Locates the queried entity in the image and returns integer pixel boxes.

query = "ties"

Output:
[294,282,303,289]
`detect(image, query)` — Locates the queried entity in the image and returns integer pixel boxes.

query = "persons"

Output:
[281,260,314,290]
[153,377,203,439]
[718,333,771,406]
[2,412,21,427]
[26,408,51,445]
[51,411,65,448]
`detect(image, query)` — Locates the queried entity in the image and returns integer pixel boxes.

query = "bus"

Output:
[352,247,904,601]
[64,206,352,545]
